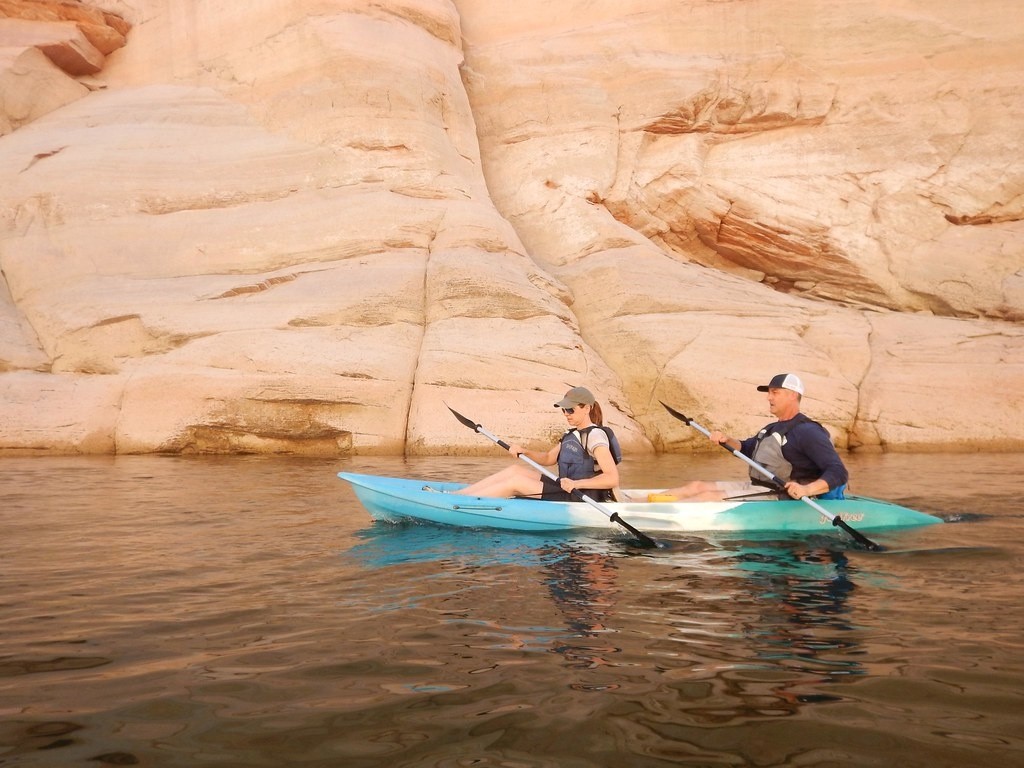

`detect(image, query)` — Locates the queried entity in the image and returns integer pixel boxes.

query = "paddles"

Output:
[656,398,880,550]
[440,398,665,548]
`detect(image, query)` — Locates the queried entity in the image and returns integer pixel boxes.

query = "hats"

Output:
[554,386,595,409]
[756,374,805,396]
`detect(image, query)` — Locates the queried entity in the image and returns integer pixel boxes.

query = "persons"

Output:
[422,387,619,502]
[630,373,849,507]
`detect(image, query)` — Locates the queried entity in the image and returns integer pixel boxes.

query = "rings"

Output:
[794,492,797,495]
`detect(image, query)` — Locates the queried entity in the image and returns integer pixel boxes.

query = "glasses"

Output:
[561,405,582,414]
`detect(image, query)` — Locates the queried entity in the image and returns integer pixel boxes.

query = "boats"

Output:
[337,471,946,539]
